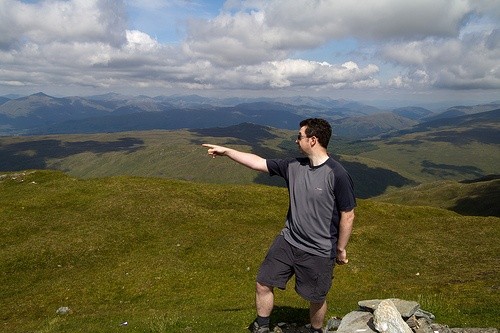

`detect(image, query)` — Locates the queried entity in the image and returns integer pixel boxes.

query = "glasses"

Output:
[297,135,306,141]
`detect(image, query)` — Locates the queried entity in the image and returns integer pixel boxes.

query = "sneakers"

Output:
[247,322,269,333]
[302,323,323,333]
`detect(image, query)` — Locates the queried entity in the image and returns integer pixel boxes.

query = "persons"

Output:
[201,118,357,333]
